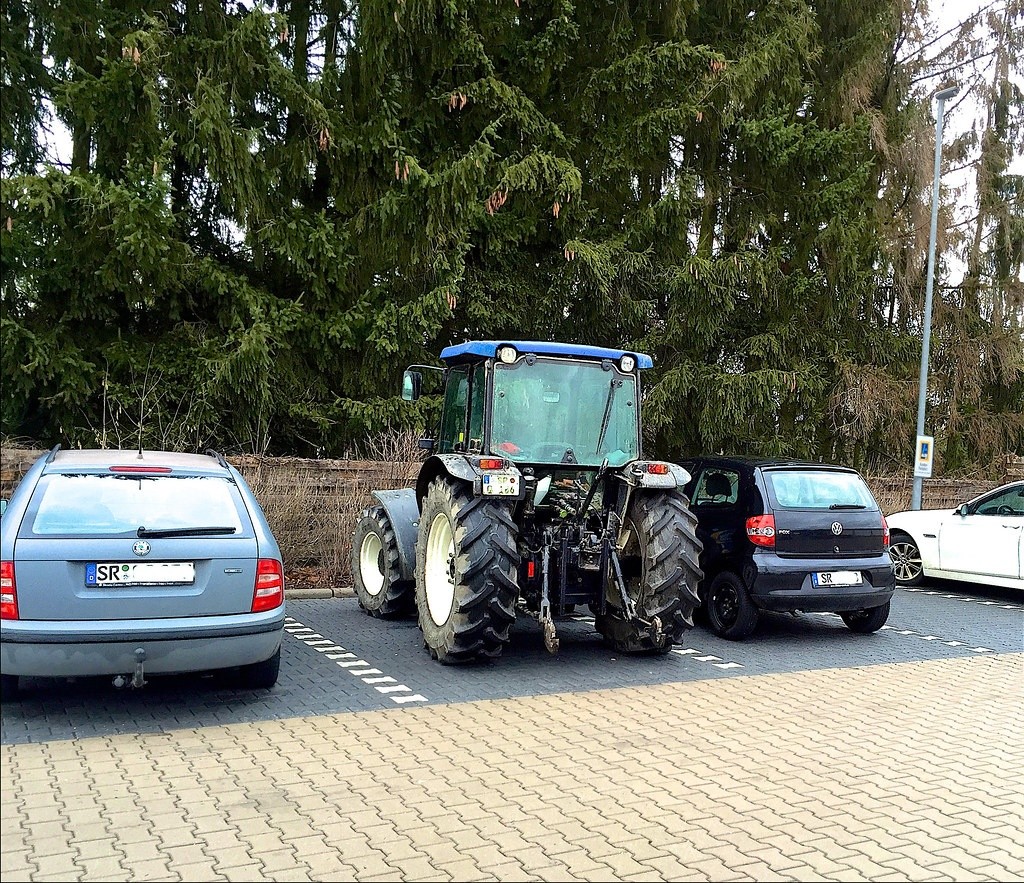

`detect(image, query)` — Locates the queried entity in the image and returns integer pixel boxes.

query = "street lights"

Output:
[910,87,960,508]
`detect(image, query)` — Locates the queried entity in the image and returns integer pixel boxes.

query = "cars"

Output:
[673,456,896,642]
[884,479,1024,592]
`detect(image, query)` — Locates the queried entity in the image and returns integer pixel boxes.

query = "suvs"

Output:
[0,443,286,694]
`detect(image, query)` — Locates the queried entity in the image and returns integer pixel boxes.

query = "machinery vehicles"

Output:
[349,339,705,668]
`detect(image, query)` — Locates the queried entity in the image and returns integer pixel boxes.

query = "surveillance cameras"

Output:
[935,86,960,100]
